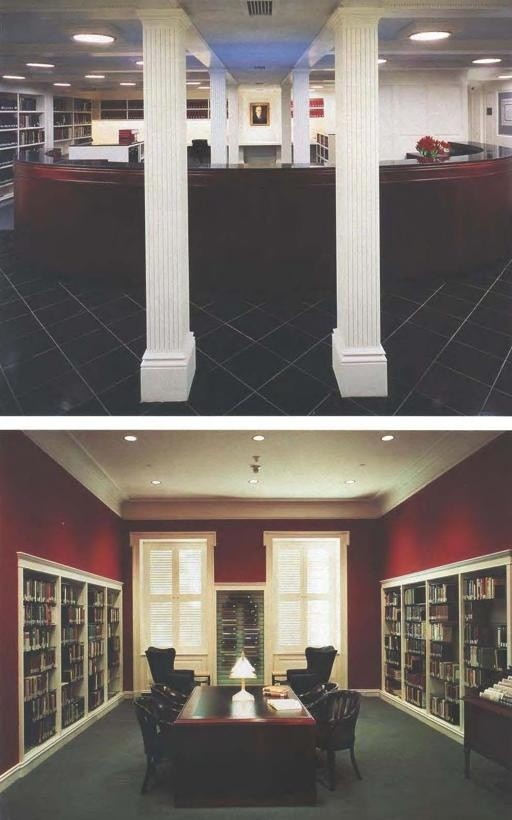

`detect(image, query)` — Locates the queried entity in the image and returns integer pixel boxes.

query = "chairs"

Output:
[138,643,362,794]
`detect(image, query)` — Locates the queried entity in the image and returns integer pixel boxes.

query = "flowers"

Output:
[417,158,450,164]
[415,137,451,158]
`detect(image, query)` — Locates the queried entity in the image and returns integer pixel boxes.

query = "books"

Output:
[384,576,512,728]
[0,98,208,197]
[24,576,121,747]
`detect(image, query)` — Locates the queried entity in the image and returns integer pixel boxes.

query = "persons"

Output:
[253,106,266,124]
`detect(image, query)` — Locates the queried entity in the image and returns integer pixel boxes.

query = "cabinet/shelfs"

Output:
[460,669,511,780]
[1,88,228,202]
[290,98,336,166]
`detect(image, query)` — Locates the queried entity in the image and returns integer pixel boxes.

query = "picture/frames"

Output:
[250,102,270,126]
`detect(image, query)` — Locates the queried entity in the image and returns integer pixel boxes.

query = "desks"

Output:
[175,685,317,807]
